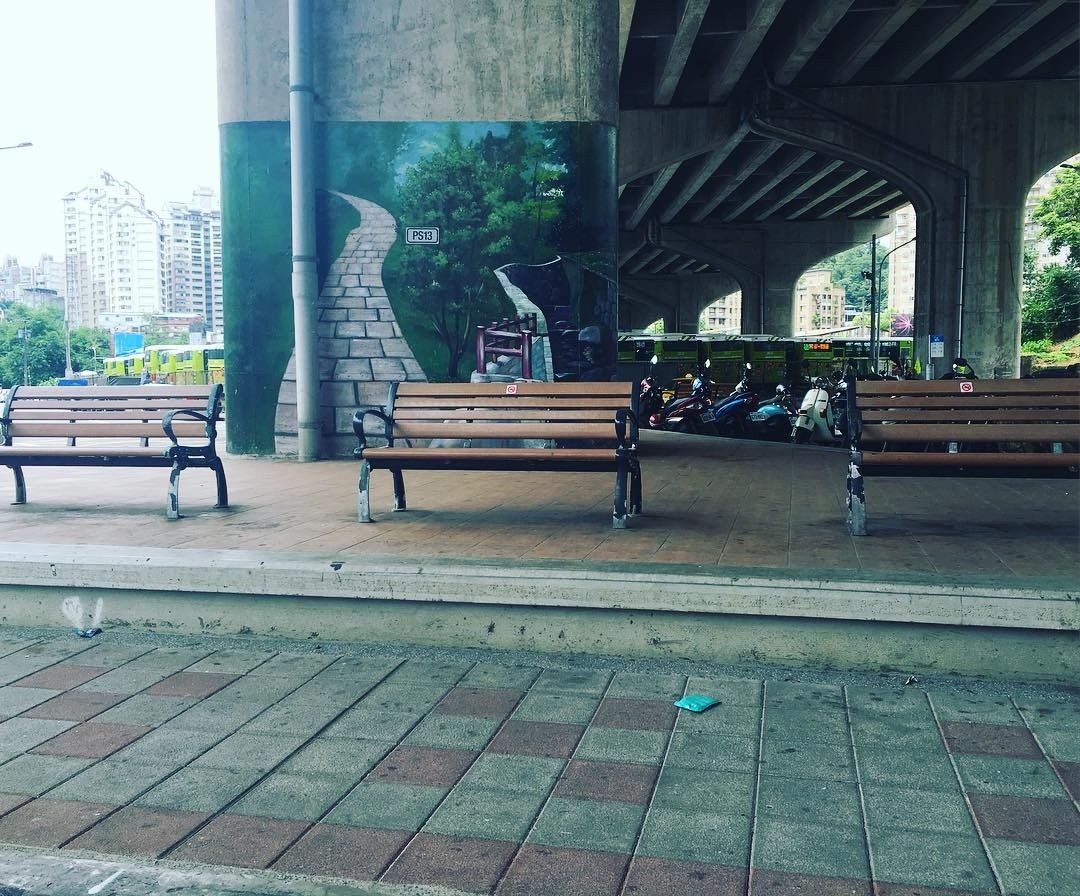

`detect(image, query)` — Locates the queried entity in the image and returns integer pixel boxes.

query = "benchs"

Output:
[351,379,645,531]
[0,383,230,521]
[844,378,1080,537]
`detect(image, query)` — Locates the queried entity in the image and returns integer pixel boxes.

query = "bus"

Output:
[618,332,914,384]
[102,345,226,421]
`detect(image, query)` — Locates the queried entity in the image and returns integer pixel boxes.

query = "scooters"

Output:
[638,355,1080,478]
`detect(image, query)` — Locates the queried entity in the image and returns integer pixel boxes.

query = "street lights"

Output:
[874,236,917,376]
